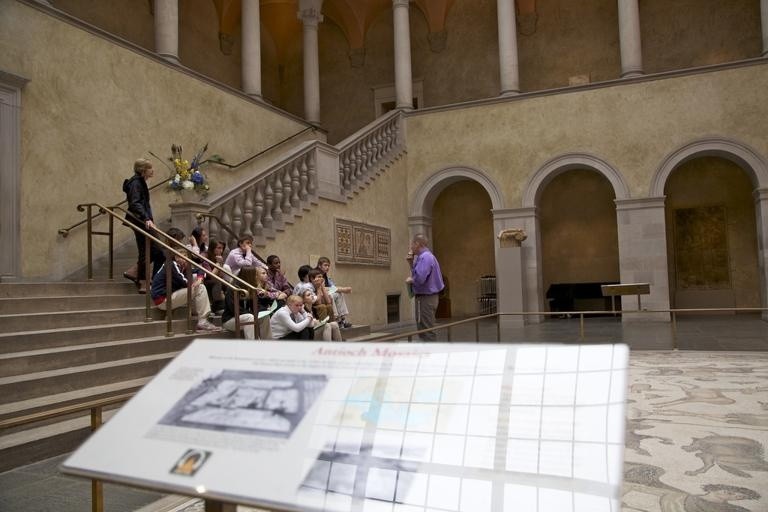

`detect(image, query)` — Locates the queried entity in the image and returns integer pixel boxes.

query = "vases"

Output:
[180,189,196,202]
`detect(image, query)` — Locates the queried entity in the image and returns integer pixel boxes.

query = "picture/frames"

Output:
[334,216,392,266]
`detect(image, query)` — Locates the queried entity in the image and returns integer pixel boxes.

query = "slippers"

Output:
[124,270,151,293]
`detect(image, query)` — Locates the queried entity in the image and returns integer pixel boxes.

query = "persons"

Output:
[405,234,445,341]
[120,158,168,293]
[151,226,354,344]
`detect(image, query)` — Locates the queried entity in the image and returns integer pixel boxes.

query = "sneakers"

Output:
[342,321,352,327]
[195,321,221,334]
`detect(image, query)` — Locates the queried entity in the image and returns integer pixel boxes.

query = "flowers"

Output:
[148,141,210,195]
